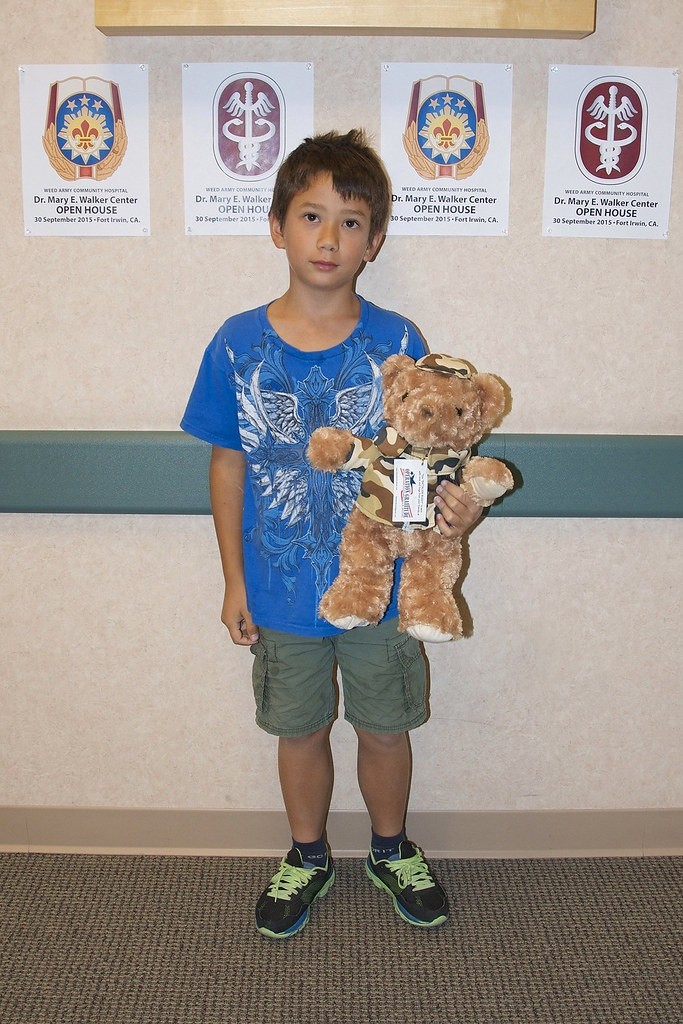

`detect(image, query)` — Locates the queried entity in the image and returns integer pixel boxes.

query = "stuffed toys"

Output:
[304,351,516,643]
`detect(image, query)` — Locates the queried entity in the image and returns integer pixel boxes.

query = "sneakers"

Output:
[365,844,451,927]
[254,846,337,938]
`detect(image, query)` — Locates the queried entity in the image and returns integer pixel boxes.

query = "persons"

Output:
[179,126,485,939]
